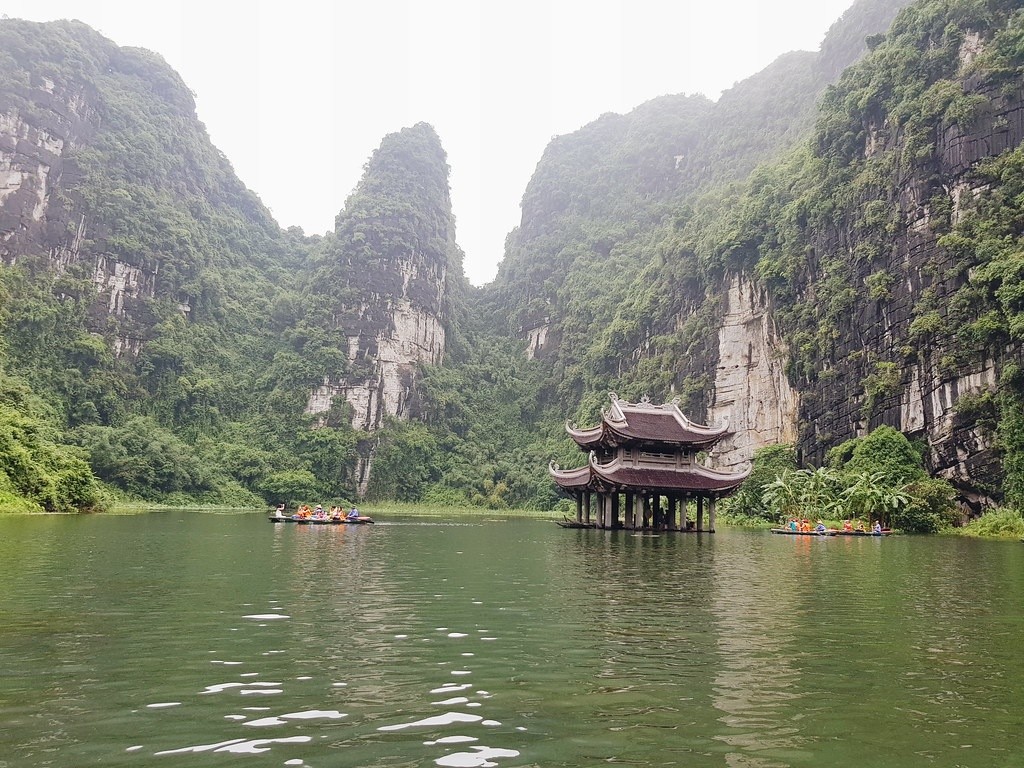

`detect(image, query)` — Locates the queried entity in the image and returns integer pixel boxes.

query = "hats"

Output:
[817,520,823,524]
[802,519,809,523]
[316,505,322,508]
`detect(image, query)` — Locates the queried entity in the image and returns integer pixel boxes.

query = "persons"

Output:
[346,506,358,521]
[660,507,669,523]
[873,521,881,532]
[858,521,865,531]
[686,509,690,525]
[814,521,825,531]
[276,504,286,517]
[298,505,326,519]
[327,506,345,520]
[844,521,852,531]
[785,518,810,531]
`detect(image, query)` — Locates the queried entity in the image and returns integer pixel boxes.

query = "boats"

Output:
[292,517,374,524]
[268,515,294,522]
[829,528,893,535]
[771,526,838,536]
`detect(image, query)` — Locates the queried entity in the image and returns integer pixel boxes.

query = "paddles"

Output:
[351,516,374,524]
